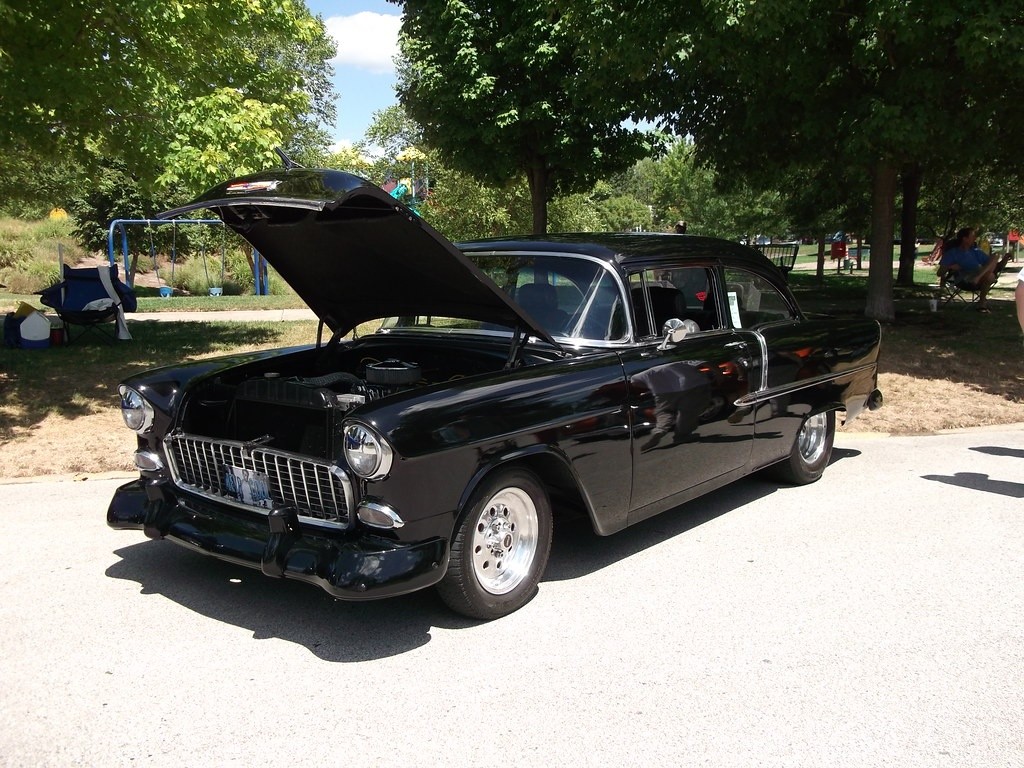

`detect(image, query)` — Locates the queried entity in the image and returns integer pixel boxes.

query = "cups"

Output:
[929,299,937,312]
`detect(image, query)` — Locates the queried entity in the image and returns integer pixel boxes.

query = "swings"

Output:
[200,225,224,297]
[148,224,175,299]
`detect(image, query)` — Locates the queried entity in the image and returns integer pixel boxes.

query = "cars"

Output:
[740,233,771,246]
[778,230,851,245]
[105,165,885,624]
[987,232,1003,246]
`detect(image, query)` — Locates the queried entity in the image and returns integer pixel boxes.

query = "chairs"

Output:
[33,264,137,347]
[517,283,569,332]
[940,238,1001,310]
[630,287,685,333]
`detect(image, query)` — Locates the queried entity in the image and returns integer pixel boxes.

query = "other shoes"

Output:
[975,299,988,311]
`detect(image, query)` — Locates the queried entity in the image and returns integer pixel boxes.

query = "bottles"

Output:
[51,329,62,344]
[19,310,51,350]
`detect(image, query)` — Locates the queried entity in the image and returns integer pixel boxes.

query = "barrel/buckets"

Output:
[3,314,21,350]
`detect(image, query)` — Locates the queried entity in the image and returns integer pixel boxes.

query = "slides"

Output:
[922,239,942,265]
[381,180,440,217]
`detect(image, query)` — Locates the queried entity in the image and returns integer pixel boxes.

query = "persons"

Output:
[1015,266,1024,333]
[427,413,505,515]
[225,467,271,508]
[630,359,711,453]
[727,343,794,424]
[937,227,1000,312]
[347,427,368,452]
[654,220,709,306]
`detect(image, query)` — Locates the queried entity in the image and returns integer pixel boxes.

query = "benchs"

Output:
[685,309,785,331]
[728,244,799,277]
